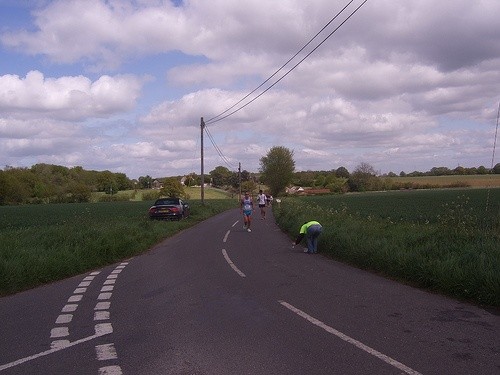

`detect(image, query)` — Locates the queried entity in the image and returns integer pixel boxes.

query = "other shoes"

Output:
[247,228,251,232]
[243,225,247,230]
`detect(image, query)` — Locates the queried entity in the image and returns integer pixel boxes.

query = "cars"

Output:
[291,186,304,192]
[149,198,190,220]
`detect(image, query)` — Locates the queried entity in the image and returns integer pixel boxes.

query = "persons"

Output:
[291,220,323,254]
[256,189,267,220]
[265,194,273,207]
[240,191,254,232]
